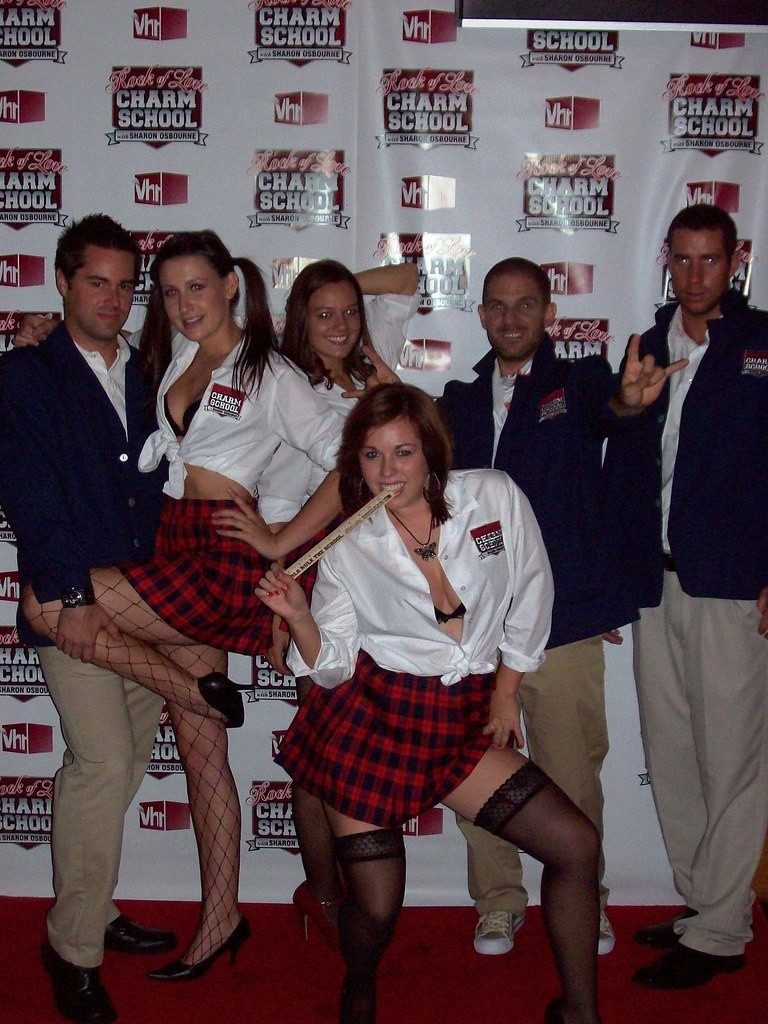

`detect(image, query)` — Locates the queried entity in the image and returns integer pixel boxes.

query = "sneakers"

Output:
[473,911,526,955]
[597,910,614,955]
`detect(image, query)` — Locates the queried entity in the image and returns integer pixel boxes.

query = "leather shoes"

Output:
[42,940,119,1023]
[631,908,747,990]
[104,914,178,955]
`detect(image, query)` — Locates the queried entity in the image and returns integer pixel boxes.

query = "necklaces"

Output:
[387,497,442,561]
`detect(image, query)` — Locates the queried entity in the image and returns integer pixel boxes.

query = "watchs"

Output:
[60,586,95,607]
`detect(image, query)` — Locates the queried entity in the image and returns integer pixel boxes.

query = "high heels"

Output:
[198,671,257,729]
[292,880,341,950]
[147,914,250,982]
[544,1000,566,1022]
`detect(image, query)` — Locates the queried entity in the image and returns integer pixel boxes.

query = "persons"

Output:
[253,380,603,1024]
[600,201,767,991]
[13,230,348,984]
[1,210,178,1024]
[254,259,424,947]
[339,256,688,955]
